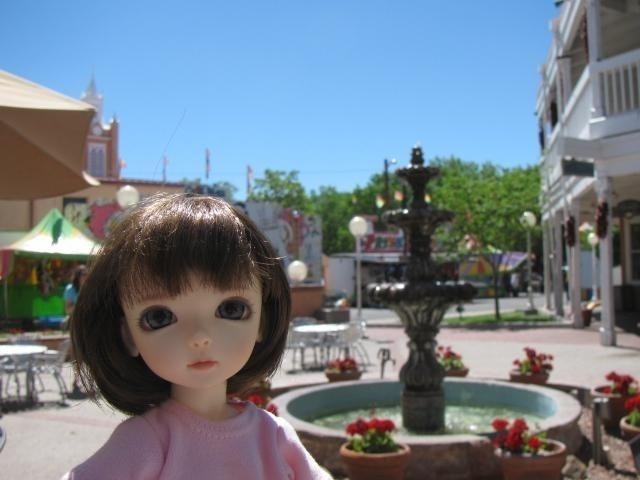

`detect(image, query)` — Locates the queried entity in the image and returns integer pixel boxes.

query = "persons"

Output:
[54,192,335,480]
[510,269,522,298]
[61,264,91,396]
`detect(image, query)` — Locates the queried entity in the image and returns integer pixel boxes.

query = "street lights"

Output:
[349,217,367,319]
[521,212,537,317]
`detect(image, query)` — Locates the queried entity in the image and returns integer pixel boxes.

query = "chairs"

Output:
[0,343,73,412]
[284,316,368,372]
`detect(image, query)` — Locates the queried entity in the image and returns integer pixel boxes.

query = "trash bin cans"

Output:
[321,310,349,323]
[583,310,593,325]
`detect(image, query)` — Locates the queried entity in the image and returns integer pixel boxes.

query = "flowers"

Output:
[436,346,464,368]
[227,390,279,417]
[600,373,635,393]
[511,347,553,374]
[488,417,554,457]
[330,359,358,372]
[625,395,639,425]
[344,418,398,454]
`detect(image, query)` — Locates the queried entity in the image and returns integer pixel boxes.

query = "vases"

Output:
[619,415,640,480]
[590,386,635,436]
[324,369,362,381]
[508,369,549,384]
[441,365,469,376]
[495,436,567,480]
[339,446,412,480]
